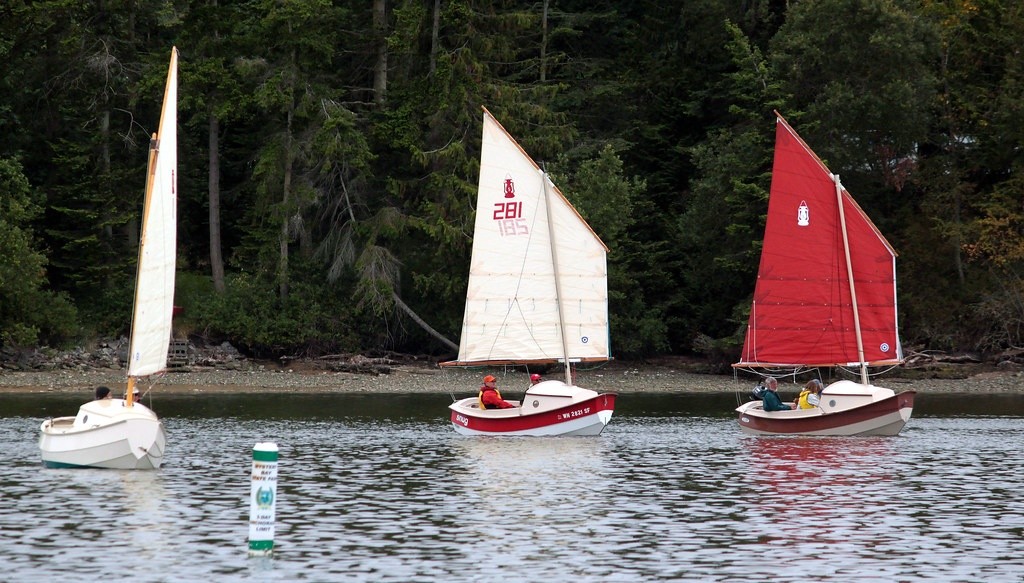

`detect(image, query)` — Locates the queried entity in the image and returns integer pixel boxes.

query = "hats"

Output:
[96,386,110,400]
[531,374,542,381]
[125,387,139,395]
[484,375,496,384]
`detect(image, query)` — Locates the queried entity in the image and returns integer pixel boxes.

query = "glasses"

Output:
[134,393,138,396]
[537,379,541,381]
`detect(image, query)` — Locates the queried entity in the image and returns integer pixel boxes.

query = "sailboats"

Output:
[39,46,178,472]
[731,108,917,438]
[438,104,620,437]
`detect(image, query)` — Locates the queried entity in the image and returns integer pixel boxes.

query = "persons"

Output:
[520,374,541,406]
[96,386,112,399]
[797,379,824,410]
[763,377,796,411]
[124,387,141,403]
[479,375,516,409]
